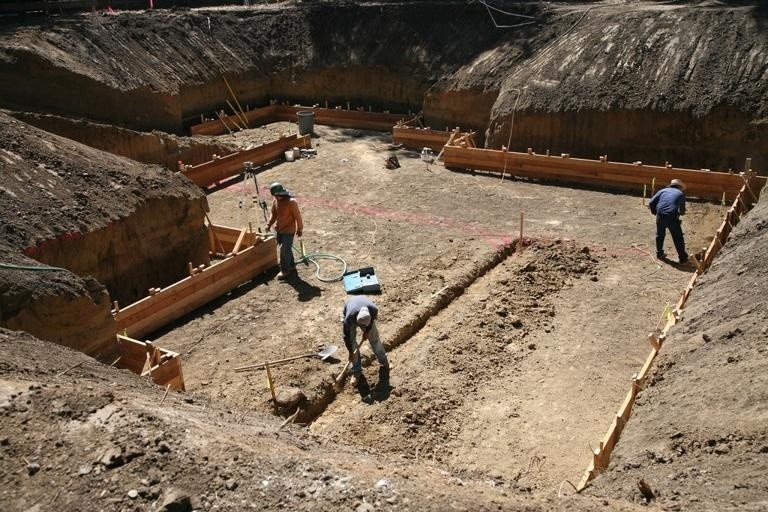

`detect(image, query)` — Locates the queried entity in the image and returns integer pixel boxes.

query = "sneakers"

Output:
[350,373,362,386]
[658,253,667,259]
[277,270,291,279]
[679,260,689,266]
[380,358,394,369]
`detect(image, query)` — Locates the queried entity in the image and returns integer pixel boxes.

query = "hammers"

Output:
[221,111,245,132]
[214,110,235,134]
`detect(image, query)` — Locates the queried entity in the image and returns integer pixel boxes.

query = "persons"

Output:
[341,294,393,388]
[263,182,304,280]
[647,178,690,266]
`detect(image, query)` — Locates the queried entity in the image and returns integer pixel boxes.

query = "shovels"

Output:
[235,345,338,372]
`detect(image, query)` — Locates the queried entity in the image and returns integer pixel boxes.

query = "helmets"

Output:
[270,183,286,195]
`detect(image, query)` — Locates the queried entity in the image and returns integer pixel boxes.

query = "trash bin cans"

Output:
[296,111,315,136]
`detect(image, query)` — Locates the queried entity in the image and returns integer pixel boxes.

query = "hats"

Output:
[666,179,686,191]
[357,307,371,327]
